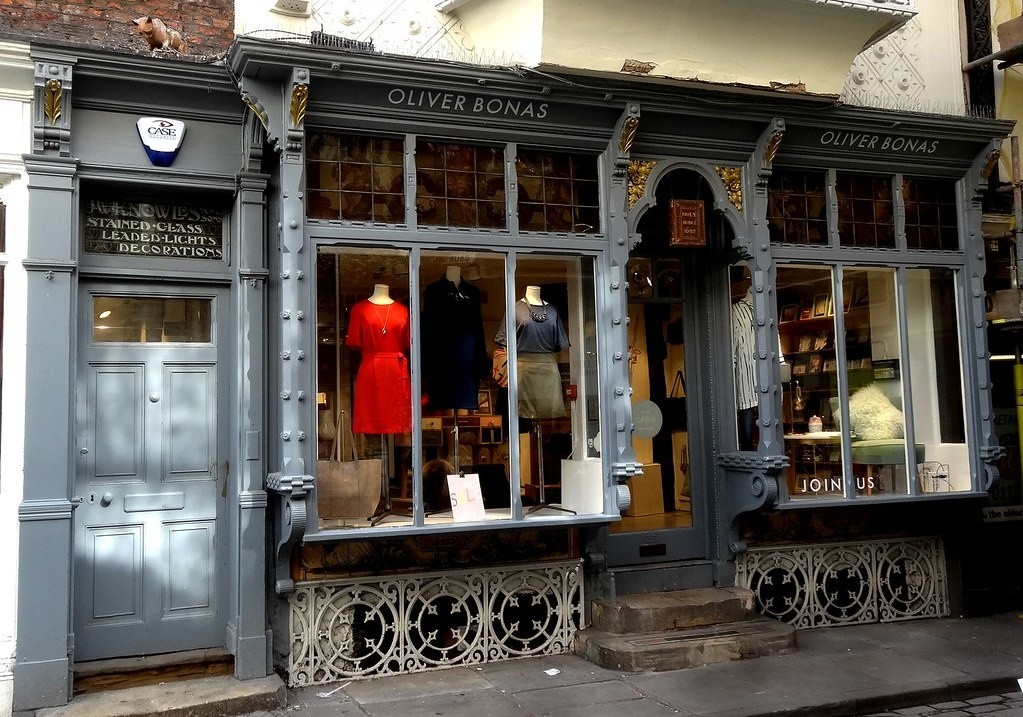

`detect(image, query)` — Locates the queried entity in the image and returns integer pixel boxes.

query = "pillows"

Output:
[832,384,904,441]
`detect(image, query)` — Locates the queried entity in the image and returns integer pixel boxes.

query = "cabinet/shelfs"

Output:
[396,415,503,447]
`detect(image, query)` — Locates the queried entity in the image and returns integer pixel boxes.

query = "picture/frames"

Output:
[473,389,492,414]
[779,281,880,495]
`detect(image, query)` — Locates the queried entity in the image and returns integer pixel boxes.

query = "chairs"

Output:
[829,396,925,496]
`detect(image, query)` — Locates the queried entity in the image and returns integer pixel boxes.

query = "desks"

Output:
[783,432,857,496]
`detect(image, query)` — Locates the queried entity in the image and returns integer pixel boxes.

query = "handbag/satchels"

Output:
[316,410,382,518]
[660,370,688,431]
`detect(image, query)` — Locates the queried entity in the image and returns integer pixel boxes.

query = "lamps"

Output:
[787,381,809,436]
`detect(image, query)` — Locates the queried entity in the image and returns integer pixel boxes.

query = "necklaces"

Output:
[373,302,390,334]
[524,296,547,322]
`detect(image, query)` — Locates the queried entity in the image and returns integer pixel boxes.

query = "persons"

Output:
[346,283,412,434]
[421,265,486,410]
[732,287,786,450]
[492,285,570,419]
